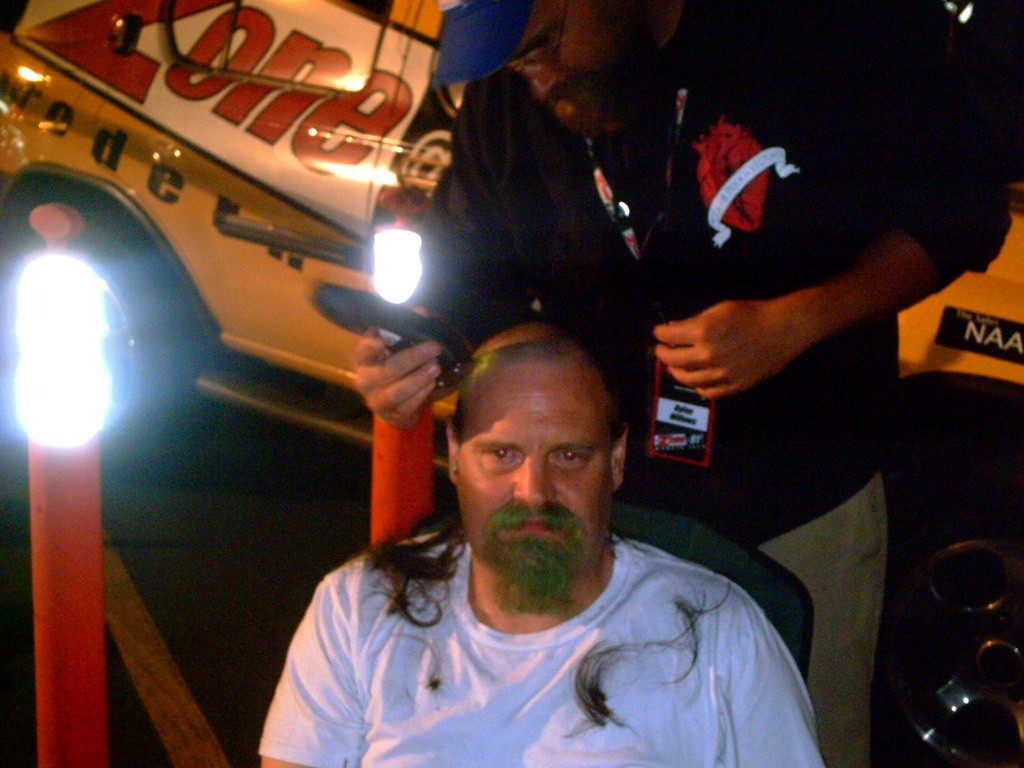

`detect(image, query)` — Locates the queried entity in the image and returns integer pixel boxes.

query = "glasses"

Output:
[504,0,569,79]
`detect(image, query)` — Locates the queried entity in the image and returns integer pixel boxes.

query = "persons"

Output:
[356,1,1015,768]
[257,325,824,768]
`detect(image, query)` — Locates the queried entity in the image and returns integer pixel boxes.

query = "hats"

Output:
[428,0,533,89]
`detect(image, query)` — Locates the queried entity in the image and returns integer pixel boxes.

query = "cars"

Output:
[1,0,1024,768]
